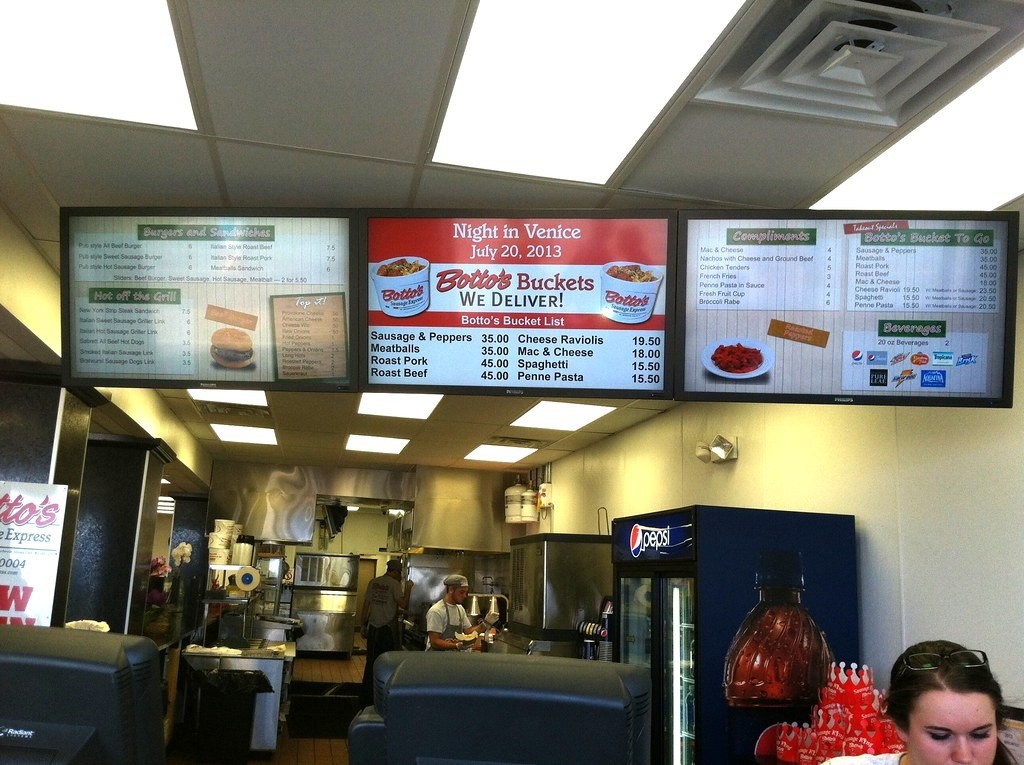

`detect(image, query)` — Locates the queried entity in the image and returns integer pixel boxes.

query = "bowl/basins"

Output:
[208,518,243,566]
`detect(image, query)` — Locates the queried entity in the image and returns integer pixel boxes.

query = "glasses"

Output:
[894,649,991,680]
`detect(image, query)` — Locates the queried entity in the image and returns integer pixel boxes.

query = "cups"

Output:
[370,256,430,317]
[598,260,663,323]
[581,639,612,661]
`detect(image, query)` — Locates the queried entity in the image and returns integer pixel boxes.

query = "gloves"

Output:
[482,610,499,629]
[456,642,474,651]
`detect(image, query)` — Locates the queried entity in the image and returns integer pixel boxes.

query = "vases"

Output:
[144,616,169,638]
[148,577,164,593]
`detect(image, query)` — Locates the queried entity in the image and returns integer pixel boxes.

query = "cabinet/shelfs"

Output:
[201,565,254,649]
[610,504,862,765]
[142,611,219,748]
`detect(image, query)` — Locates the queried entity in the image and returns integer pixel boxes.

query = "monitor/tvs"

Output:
[60,204,1018,410]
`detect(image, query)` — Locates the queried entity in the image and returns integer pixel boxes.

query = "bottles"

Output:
[725,547,833,765]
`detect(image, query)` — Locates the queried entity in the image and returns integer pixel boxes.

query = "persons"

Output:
[425,575,500,651]
[359,559,414,697]
[816,642,1023,765]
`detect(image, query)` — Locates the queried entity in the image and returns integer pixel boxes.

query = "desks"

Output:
[182,640,296,750]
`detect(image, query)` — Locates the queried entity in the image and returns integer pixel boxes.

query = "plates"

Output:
[701,337,774,379]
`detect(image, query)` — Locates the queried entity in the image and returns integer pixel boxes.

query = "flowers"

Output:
[146,589,168,625]
[168,542,192,604]
[149,555,170,577]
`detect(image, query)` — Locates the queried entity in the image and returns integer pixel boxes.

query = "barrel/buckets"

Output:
[190,669,275,764]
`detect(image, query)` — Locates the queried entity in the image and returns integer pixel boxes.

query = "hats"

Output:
[387,560,402,572]
[444,575,468,587]
